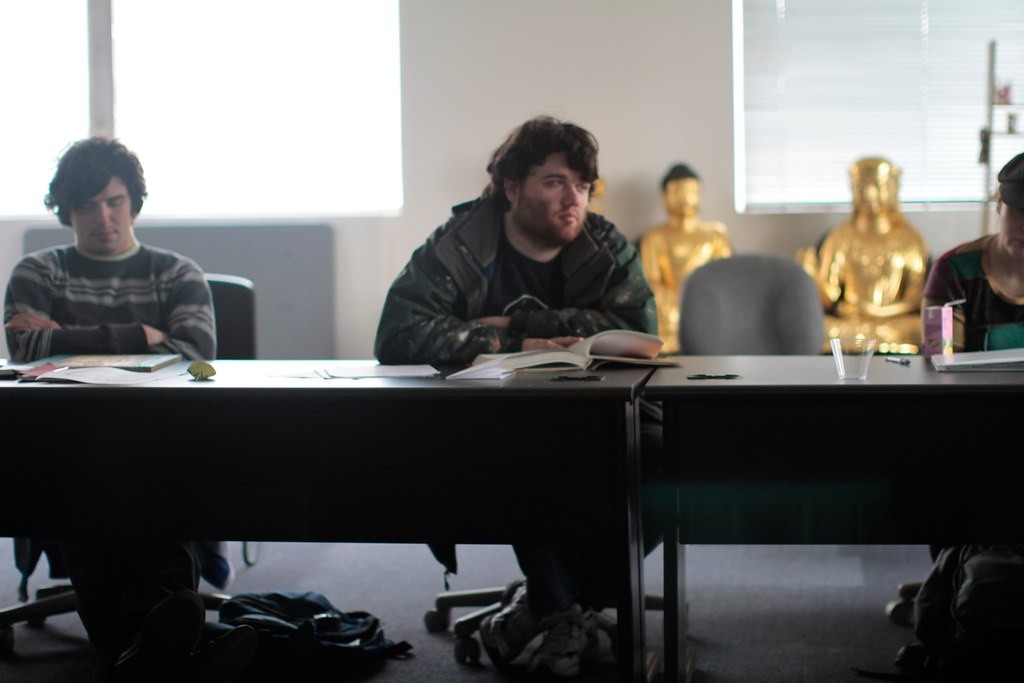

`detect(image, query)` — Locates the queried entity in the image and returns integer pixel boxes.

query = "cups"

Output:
[829,337,877,381]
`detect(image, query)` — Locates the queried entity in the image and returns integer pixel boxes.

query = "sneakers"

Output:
[527,601,601,680]
[479,585,544,668]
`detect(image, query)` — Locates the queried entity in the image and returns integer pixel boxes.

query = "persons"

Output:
[643,165,733,352]
[920,154,1023,683]
[796,156,931,352]
[5,136,217,670]
[377,115,659,683]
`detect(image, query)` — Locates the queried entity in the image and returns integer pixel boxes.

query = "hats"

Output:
[997,152,1024,207]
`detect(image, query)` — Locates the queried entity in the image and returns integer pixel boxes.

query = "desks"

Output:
[645,354,1024,683]
[0,358,662,683]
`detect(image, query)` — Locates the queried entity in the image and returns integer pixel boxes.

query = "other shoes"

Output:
[114,590,258,683]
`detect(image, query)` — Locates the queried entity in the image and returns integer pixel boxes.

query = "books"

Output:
[438,328,679,379]
[928,348,1024,371]
[2,354,183,380]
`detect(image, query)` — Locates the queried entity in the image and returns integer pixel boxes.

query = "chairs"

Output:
[680,256,825,355]
[0,273,255,654]
